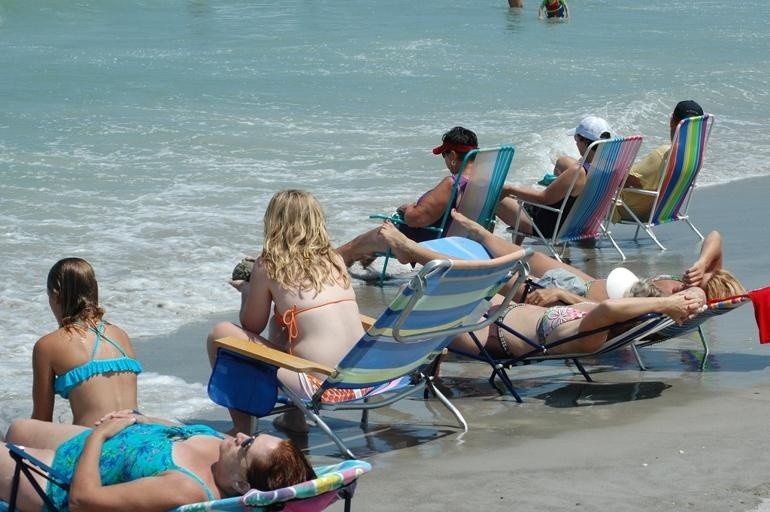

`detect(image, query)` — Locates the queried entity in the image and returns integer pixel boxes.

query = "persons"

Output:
[444,287,707,358]
[538,0,569,19]
[207,189,366,437]
[379,208,744,308]
[496,116,621,239]
[508,0,523,9]
[553,100,705,225]
[0,409,317,512]
[333,127,490,268]
[31,258,142,428]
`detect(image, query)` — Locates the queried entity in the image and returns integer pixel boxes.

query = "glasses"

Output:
[241,433,259,470]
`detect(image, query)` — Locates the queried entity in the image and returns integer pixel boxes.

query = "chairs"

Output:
[1,442,372,512]
[211,246,535,460]
[607,113,715,250]
[506,134,643,264]
[436,283,708,404]
[630,286,769,371]
[370,145,515,287]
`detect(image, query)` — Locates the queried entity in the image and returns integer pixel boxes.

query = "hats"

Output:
[606,267,638,299]
[673,100,703,117]
[566,117,614,141]
[432,142,479,154]
